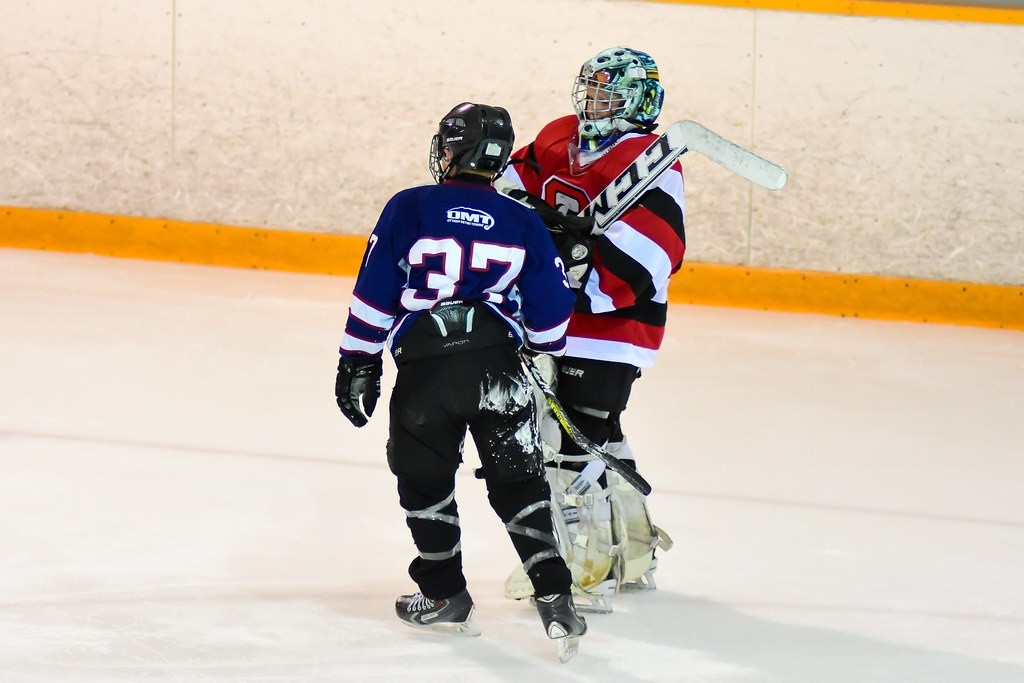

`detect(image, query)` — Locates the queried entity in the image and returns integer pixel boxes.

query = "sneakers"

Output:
[535,594,586,663]
[396,588,481,636]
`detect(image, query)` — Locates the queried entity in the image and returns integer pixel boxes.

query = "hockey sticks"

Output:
[519,349,653,497]
[578,119,787,237]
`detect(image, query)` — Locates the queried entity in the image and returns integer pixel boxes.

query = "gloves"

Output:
[336,358,384,428]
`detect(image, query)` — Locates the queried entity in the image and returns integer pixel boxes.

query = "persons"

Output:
[334,102,591,663]
[492,46,687,615]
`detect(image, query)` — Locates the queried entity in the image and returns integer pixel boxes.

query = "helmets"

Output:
[572,47,664,151]
[429,102,514,184]
